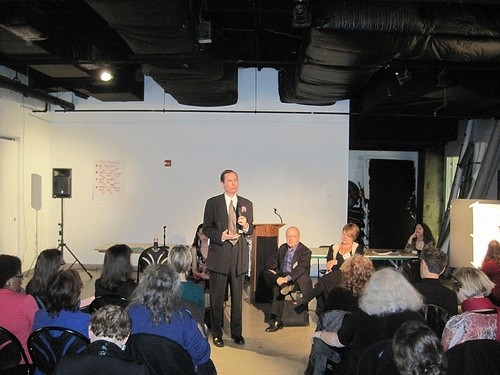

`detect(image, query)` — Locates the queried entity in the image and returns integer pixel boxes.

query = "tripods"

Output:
[56,197,94,278]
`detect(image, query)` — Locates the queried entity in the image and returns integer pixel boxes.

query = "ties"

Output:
[228,200,240,246]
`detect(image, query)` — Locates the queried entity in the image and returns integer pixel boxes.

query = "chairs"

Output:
[321,287,500,375]
[136,245,169,284]
[0,295,197,375]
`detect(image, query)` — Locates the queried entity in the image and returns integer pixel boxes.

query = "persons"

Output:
[25,248,65,298]
[167,244,205,321]
[440,267,500,352]
[95,244,136,301]
[478,240,500,307]
[320,254,374,315]
[391,320,500,375]
[122,265,218,375]
[404,222,435,281]
[32,270,94,375]
[54,304,157,375]
[186,224,211,325]
[0,254,40,365]
[202,170,254,347]
[409,241,458,315]
[337,268,429,375]
[263,227,314,332]
[292,222,364,315]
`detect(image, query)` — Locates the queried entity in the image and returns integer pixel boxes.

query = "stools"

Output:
[274,286,310,327]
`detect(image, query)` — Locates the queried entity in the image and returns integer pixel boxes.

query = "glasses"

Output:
[15,274,24,279]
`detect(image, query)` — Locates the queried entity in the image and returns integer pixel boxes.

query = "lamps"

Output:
[86,65,145,101]
[196,22,212,43]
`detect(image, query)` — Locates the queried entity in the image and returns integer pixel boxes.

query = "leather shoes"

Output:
[280,283,294,295]
[213,336,224,347]
[266,320,283,332]
[231,336,245,345]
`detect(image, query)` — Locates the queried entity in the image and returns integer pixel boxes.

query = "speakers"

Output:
[52,168,72,198]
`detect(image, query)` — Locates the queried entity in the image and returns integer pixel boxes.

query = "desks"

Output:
[310,246,419,281]
[97,242,175,254]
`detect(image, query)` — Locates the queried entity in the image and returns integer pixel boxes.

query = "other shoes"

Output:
[293,298,308,308]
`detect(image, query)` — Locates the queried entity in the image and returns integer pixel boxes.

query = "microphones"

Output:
[272,208,283,223]
